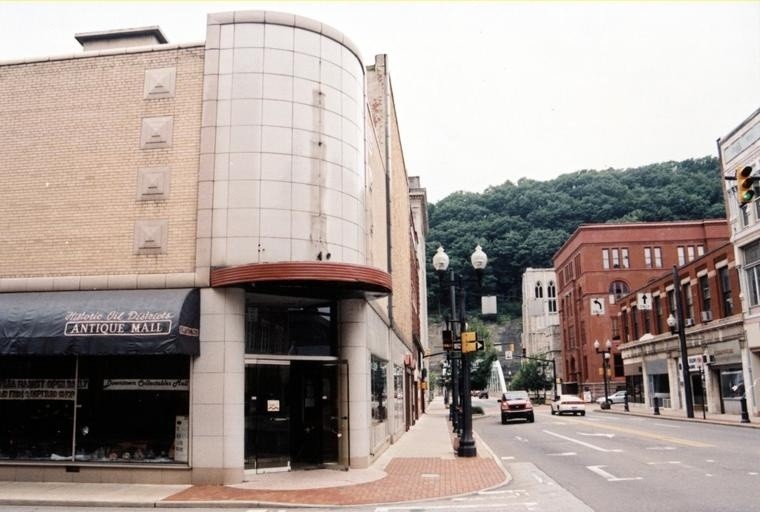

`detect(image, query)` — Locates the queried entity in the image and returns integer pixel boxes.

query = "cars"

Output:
[471,388,488,400]
[596,389,627,404]
[550,394,586,416]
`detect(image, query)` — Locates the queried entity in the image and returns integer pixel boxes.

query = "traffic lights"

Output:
[736,166,755,203]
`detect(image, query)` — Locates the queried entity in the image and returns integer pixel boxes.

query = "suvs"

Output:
[496,391,535,424]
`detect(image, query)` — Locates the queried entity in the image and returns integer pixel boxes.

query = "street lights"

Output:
[594,339,611,409]
[432,244,488,458]
[663,265,694,419]
